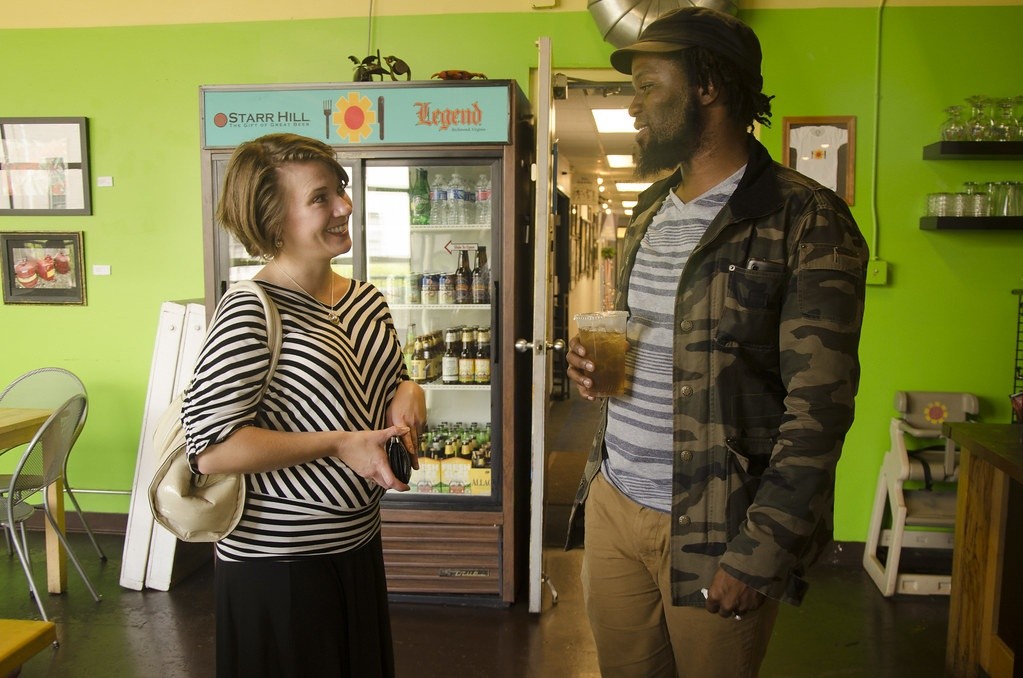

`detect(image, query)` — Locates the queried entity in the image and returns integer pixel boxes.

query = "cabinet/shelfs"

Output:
[552,292,571,401]
[918,141,1023,230]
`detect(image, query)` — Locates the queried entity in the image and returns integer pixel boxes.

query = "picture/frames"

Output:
[781,114,855,204]
[0,116,92,216]
[570,203,598,288]
[0,231,88,305]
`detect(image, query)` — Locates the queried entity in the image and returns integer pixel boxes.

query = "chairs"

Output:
[0,395,103,650]
[861,389,982,597]
[0,367,107,598]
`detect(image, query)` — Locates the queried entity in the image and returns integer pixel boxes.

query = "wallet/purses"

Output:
[388,435,411,484]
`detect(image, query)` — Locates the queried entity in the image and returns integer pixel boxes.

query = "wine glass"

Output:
[966,96,993,141]
[940,105,967,141]
[993,95,1023,140]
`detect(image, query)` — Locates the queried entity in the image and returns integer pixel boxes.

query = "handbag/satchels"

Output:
[148,280,283,542]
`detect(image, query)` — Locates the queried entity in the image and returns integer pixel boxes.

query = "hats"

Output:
[611,7,763,90]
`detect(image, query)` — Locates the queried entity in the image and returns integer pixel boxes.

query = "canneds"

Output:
[387,271,457,306]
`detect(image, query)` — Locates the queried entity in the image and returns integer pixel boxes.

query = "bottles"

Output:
[419,421,491,468]
[455,245,489,303]
[411,167,492,224]
[403,322,491,386]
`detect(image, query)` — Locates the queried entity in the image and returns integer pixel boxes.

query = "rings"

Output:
[734,615,741,621]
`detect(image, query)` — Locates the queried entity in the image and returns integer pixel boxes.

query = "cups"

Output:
[926,180,1023,216]
[575,310,631,398]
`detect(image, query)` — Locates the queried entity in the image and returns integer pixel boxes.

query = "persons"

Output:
[566,8,870,678]
[180,133,428,678]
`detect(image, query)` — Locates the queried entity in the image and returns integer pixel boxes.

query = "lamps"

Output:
[597,175,613,217]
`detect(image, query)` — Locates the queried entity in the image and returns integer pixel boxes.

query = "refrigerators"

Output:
[198,78,532,606]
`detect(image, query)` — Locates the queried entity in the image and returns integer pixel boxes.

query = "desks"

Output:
[0,619,58,678]
[0,409,73,592]
[938,419,1023,678]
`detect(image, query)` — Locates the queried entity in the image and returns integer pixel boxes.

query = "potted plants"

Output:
[601,247,616,261]
